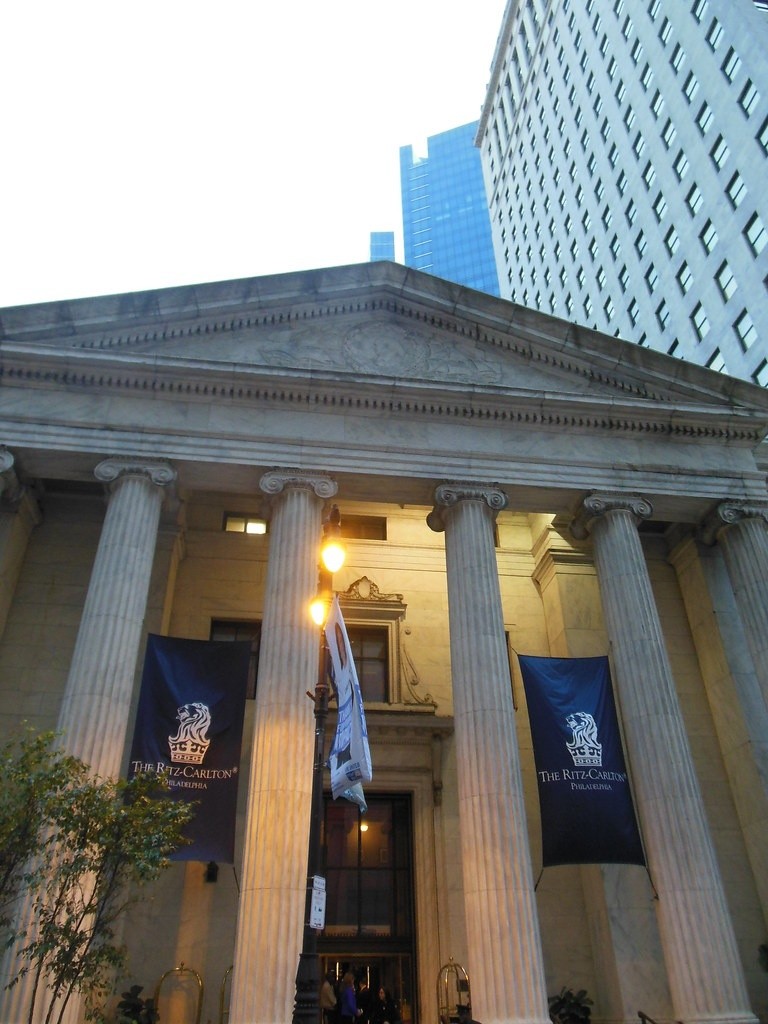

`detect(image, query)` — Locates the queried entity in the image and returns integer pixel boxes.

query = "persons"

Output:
[322,965,396,1024]
[335,623,347,671]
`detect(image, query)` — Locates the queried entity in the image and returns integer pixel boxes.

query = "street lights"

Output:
[291,504,344,1024]
